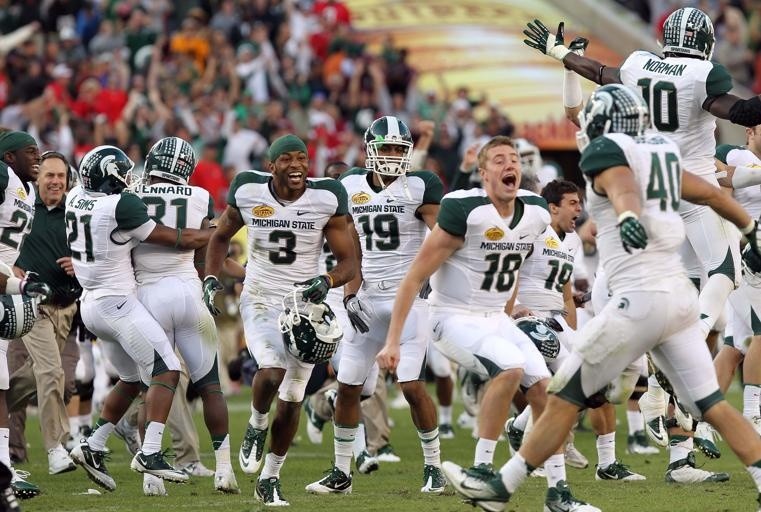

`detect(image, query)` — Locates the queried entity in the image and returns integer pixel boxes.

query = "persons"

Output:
[0,272,53,510]
[71,144,192,491]
[128,137,243,498]
[441,83,761,511]
[1,130,42,499]
[668,387,730,483]
[3,1,761,476]
[377,135,603,511]
[205,134,358,506]
[506,180,648,481]
[307,116,447,497]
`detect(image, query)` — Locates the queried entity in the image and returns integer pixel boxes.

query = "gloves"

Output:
[745,219,761,256]
[294,276,330,304]
[524,19,589,69]
[23,281,52,305]
[202,275,224,316]
[343,296,372,333]
[615,215,648,254]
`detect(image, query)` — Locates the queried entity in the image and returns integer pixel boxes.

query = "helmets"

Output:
[278,286,344,364]
[364,116,414,176]
[0,294,37,340]
[575,84,650,154]
[513,316,560,367]
[662,7,717,61]
[78,136,196,193]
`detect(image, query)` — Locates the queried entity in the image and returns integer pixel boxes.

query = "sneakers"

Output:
[0,395,760,512]
